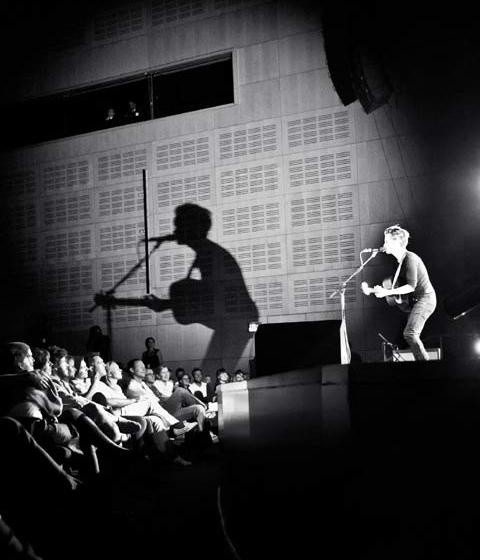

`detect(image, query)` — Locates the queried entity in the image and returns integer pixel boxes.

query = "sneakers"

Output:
[116,417,217,467]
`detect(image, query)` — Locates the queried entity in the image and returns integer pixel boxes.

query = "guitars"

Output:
[361,277,413,314]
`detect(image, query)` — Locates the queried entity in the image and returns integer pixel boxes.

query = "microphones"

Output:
[363,247,385,252]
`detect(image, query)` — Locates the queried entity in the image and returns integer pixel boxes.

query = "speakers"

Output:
[320,0,415,106]
[392,348,442,362]
[254,320,352,376]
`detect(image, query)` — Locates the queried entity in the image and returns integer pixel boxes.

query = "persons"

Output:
[362,225,437,361]
